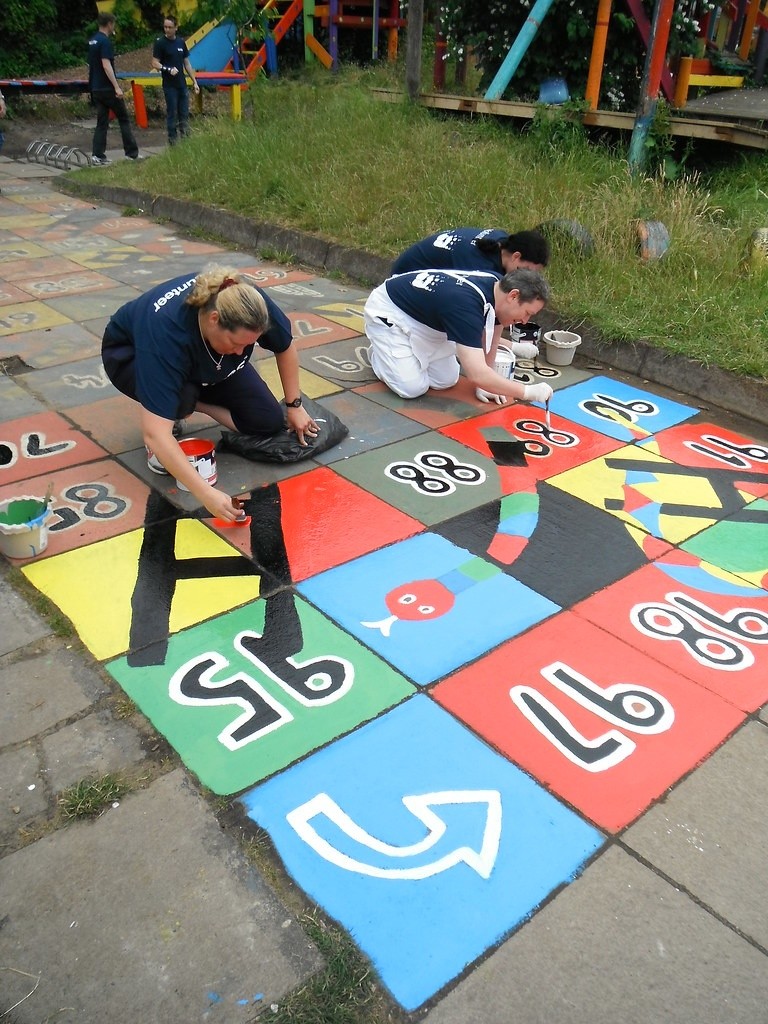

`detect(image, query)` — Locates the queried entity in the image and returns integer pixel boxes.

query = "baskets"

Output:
[495,344,516,381]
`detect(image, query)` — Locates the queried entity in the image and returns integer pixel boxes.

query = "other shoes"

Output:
[92,156,112,166]
[125,155,143,160]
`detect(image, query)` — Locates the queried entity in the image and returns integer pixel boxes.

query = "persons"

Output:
[87,11,150,166]
[151,14,200,151]
[101,260,321,523]
[390,228,550,359]
[364,268,553,404]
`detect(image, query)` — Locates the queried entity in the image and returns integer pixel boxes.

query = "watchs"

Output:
[285,396,302,408]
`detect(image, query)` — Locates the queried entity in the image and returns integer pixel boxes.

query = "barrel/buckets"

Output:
[176,437,218,492]
[0,495,54,559]
[493,344,516,381]
[512,321,542,349]
[542,330,582,367]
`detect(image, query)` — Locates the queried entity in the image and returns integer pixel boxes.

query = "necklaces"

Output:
[198,306,225,371]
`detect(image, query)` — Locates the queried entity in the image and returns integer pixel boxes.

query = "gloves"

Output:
[511,342,539,359]
[522,381,553,403]
[476,387,507,404]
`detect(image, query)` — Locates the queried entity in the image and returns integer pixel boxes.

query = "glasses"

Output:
[164,25,174,29]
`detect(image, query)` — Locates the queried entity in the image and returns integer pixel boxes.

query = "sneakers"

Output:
[172,419,188,436]
[144,444,169,474]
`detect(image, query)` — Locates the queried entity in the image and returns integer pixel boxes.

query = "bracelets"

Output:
[160,64,172,73]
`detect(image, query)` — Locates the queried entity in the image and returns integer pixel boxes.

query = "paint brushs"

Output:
[545,399,552,430]
[532,339,538,362]
[231,497,248,524]
